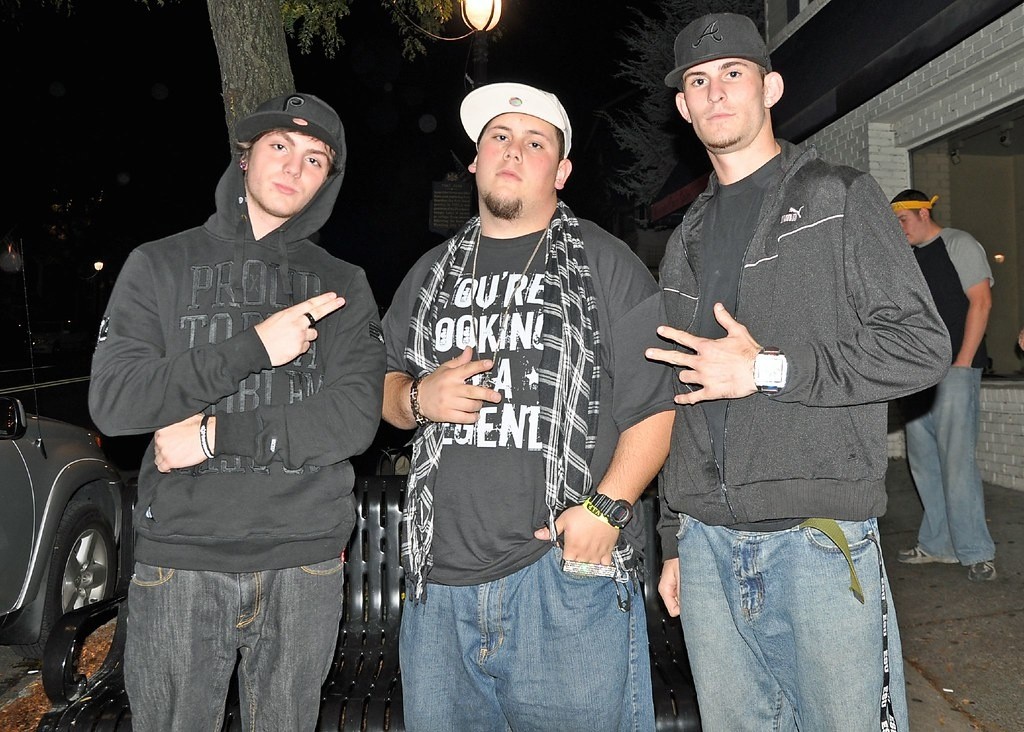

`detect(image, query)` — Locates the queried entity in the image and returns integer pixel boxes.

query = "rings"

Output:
[304,312,315,326]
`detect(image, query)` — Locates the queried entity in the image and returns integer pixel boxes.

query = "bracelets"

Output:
[411,376,430,427]
[583,497,619,529]
[200,413,214,459]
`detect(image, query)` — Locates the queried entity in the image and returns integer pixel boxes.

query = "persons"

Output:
[655,13,954,732]
[383,82,677,732]
[89,93,387,732]
[888,190,998,581]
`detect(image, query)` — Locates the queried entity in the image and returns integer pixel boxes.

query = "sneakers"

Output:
[969,559,997,580]
[896,546,937,565]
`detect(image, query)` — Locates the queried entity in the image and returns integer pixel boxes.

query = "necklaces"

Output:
[470,227,550,388]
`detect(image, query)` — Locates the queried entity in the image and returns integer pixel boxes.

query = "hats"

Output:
[460,82,571,159]
[664,14,771,89]
[236,93,341,161]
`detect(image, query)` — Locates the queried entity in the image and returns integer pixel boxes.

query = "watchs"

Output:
[589,491,634,528]
[753,346,787,398]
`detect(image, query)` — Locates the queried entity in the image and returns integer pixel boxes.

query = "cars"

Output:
[0,394,126,661]
